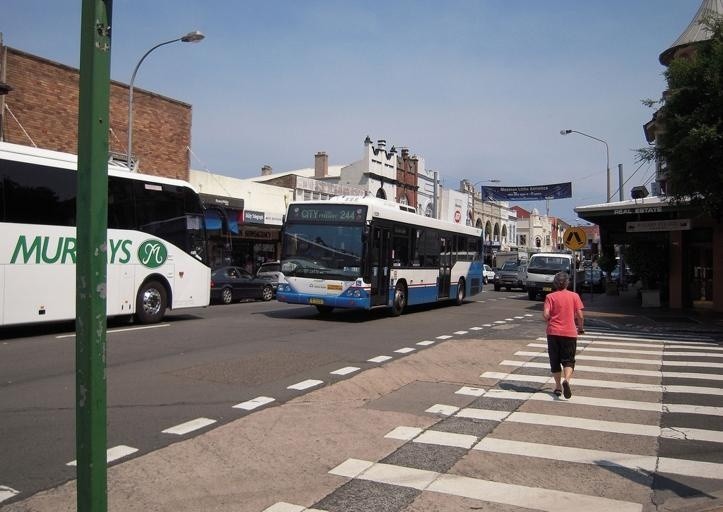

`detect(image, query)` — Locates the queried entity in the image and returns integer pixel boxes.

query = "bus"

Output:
[279,195,484,316]
[0,138,228,329]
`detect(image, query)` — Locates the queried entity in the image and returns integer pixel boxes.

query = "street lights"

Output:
[561,129,611,281]
[469,179,499,225]
[126,32,207,168]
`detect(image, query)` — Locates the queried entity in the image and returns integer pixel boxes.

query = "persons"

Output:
[544,271,585,399]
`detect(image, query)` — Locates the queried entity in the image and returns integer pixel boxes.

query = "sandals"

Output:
[554,380,571,399]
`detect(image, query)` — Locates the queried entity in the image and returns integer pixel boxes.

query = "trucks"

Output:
[526,255,586,298]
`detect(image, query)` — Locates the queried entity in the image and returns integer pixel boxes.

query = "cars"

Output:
[585,257,633,292]
[482,264,500,285]
[210,262,280,301]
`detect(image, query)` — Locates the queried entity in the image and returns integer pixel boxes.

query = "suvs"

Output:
[493,260,527,292]
